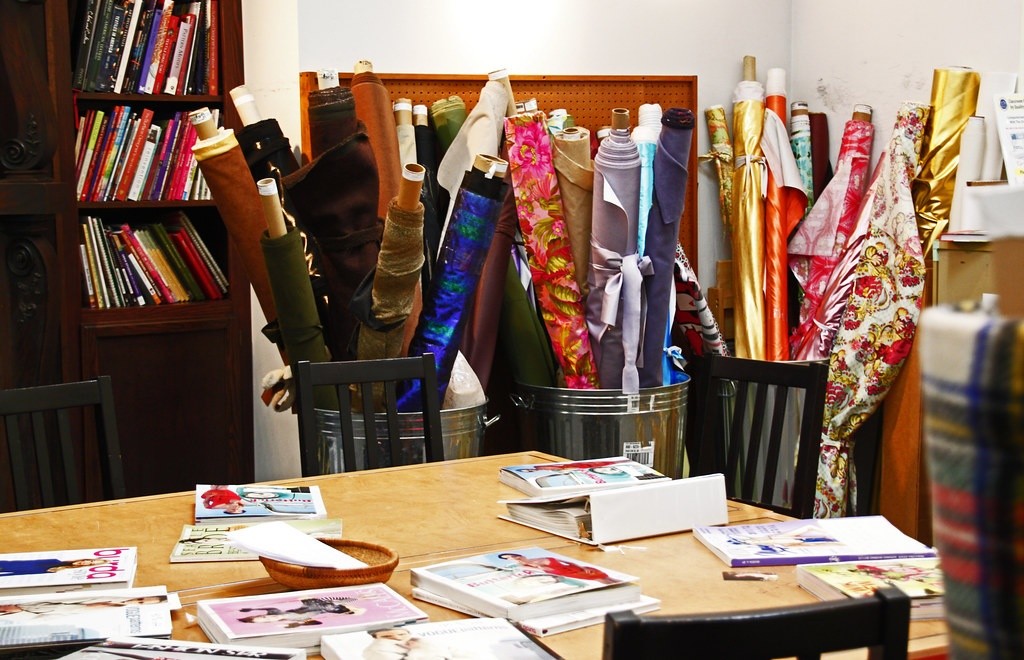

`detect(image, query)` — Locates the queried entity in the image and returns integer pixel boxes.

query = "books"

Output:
[73,0,230,308]
[169,484,369,570]
[197,581,430,655]
[320,617,563,660]
[0,546,174,651]
[500,456,729,546]
[59,635,306,660]
[409,544,661,638]
[692,516,948,619]
[993,92,1024,185]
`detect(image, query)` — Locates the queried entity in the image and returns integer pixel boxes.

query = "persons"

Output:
[517,459,632,473]
[728,525,842,547]
[848,565,941,584]
[178,534,233,548]
[236,596,358,628]
[0,593,167,624]
[498,553,621,584]
[364,628,446,660]
[200,485,246,515]
[0,558,110,577]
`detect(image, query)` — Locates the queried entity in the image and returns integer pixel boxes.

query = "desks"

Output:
[0,450,938,660]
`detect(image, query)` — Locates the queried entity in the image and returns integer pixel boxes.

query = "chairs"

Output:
[0,374,127,515]
[293,354,445,478]
[697,353,828,519]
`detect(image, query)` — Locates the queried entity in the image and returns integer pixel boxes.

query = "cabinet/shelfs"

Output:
[1,1,255,509]
